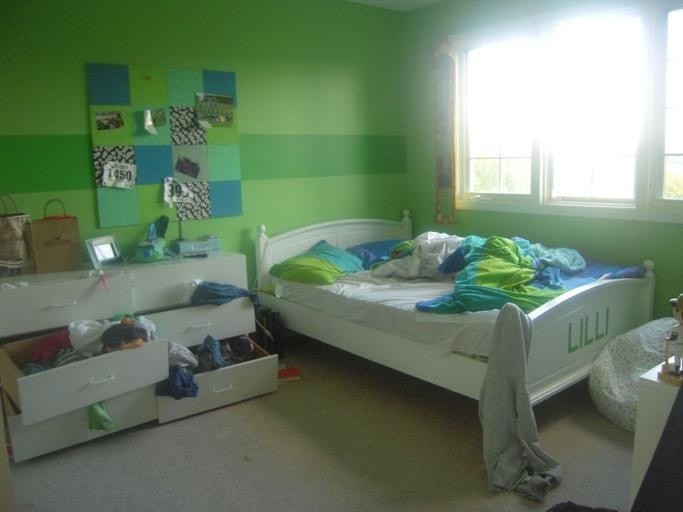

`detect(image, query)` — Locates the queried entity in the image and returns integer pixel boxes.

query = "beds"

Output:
[253,208,654,446]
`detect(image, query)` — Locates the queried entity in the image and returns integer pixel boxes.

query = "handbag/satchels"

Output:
[0,192,33,278]
[19,197,85,273]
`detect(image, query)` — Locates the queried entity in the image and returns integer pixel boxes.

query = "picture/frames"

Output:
[84,234,126,270]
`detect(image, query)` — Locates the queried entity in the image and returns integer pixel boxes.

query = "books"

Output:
[277,368,301,383]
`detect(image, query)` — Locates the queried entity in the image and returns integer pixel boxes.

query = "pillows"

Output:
[269,240,407,286]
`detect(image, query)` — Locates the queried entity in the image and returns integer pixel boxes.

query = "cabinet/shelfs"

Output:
[0,247,279,464]
